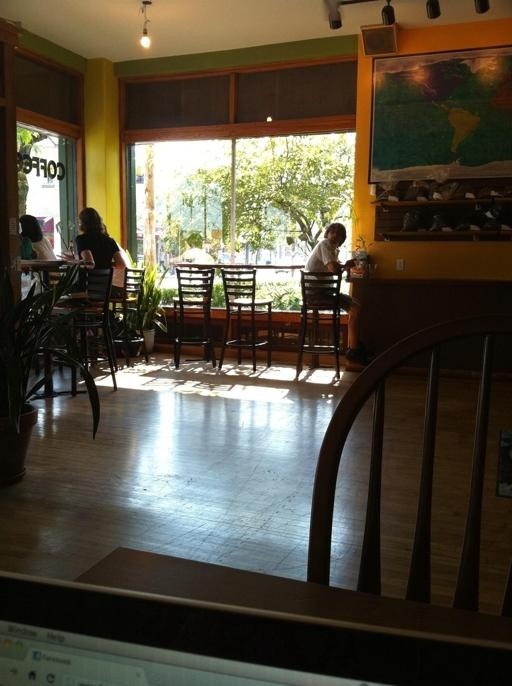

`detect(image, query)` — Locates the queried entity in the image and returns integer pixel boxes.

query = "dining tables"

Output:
[0,261,100,482]
[113,307,144,358]
[128,258,167,353]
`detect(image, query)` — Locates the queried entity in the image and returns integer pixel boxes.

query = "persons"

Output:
[169,234,215,288]
[110,235,134,300]
[20,215,60,284]
[75,207,125,300]
[305,224,369,367]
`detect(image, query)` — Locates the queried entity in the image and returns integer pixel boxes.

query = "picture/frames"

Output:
[72,544,511,642]
[174,264,309,269]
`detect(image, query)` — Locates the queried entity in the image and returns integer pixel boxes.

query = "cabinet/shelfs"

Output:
[356,248,369,279]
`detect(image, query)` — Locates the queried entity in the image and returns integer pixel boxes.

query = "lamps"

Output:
[61,267,118,396]
[172,267,218,370]
[308,311,511,620]
[296,269,343,379]
[218,268,272,373]
[111,267,149,366]
[45,268,74,307]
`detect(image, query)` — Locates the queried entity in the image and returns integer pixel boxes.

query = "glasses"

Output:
[381,0,396,26]
[475,0,490,14]
[426,1,441,19]
[140,4,150,49]
[326,0,343,29]
